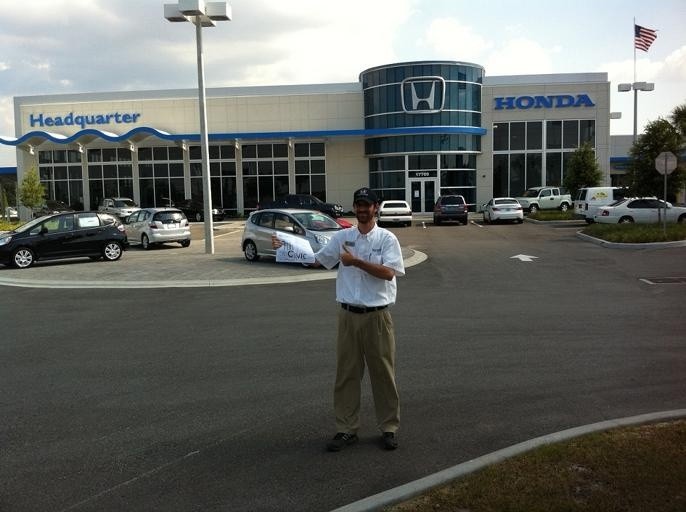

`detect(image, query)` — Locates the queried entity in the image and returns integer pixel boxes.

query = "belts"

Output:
[342,303,387,313]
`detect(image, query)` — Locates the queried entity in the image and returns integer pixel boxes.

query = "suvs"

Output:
[99,197,142,219]
[433,194,468,226]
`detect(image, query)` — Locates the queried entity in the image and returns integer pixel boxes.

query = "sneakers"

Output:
[327,433,358,450]
[384,434,397,450]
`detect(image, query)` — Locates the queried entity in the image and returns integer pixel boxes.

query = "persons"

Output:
[270,186,405,452]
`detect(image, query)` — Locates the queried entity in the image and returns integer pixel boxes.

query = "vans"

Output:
[573,186,658,224]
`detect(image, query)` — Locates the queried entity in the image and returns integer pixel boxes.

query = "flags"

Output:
[634,24,659,52]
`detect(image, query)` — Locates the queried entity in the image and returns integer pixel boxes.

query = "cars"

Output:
[594,195,686,224]
[482,197,524,224]
[0,211,127,269]
[174,200,226,222]
[241,209,345,269]
[118,207,193,249]
[257,194,344,219]
[35,200,76,218]
[377,200,412,227]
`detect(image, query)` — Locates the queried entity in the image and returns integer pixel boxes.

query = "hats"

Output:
[354,187,377,204]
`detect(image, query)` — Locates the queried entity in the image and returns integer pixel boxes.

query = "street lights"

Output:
[617,81,655,183]
[163,0,233,255]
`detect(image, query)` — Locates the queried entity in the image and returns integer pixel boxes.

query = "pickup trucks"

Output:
[517,186,572,215]
